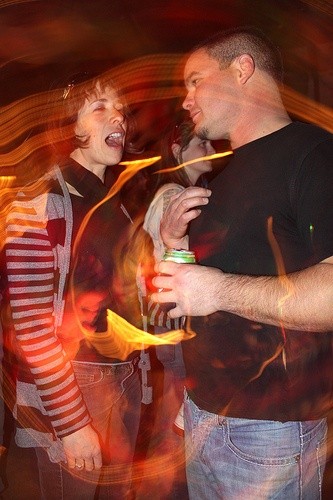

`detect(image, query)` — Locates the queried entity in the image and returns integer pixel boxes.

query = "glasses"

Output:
[60,71,91,99]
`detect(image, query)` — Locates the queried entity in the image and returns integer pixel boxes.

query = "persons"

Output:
[47,250,109,356]
[148,29,333,500]
[1,66,157,500]
[136,111,222,448]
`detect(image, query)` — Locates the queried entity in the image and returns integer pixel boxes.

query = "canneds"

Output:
[163,249,196,269]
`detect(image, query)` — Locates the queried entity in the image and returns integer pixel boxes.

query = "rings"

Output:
[75,462,85,468]
